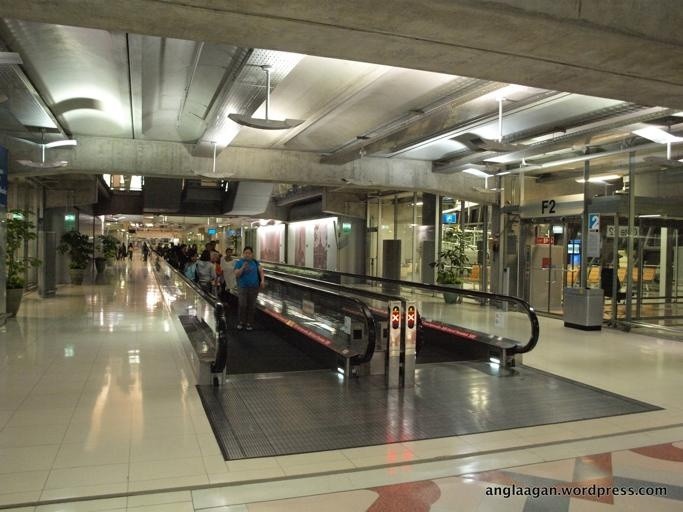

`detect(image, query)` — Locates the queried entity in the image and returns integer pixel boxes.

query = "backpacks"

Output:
[191,262,201,284]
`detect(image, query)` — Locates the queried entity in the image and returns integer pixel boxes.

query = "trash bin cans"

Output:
[563,286,604,331]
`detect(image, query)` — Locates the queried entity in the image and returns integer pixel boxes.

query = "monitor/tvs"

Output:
[553,226,562,234]
[442,213,458,224]
[568,243,579,254]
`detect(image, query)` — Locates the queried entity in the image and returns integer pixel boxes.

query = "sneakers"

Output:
[237,321,243,330]
[246,323,253,331]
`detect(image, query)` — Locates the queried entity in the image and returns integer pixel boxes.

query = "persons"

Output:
[115,241,265,331]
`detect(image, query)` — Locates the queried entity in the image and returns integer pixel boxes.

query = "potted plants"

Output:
[423,227,476,306]
[0,202,122,320]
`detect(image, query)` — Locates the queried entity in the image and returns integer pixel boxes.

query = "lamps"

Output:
[192,142,236,179]
[11,128,68,170]
[226,62,306,132]
[444,94,683,186]
